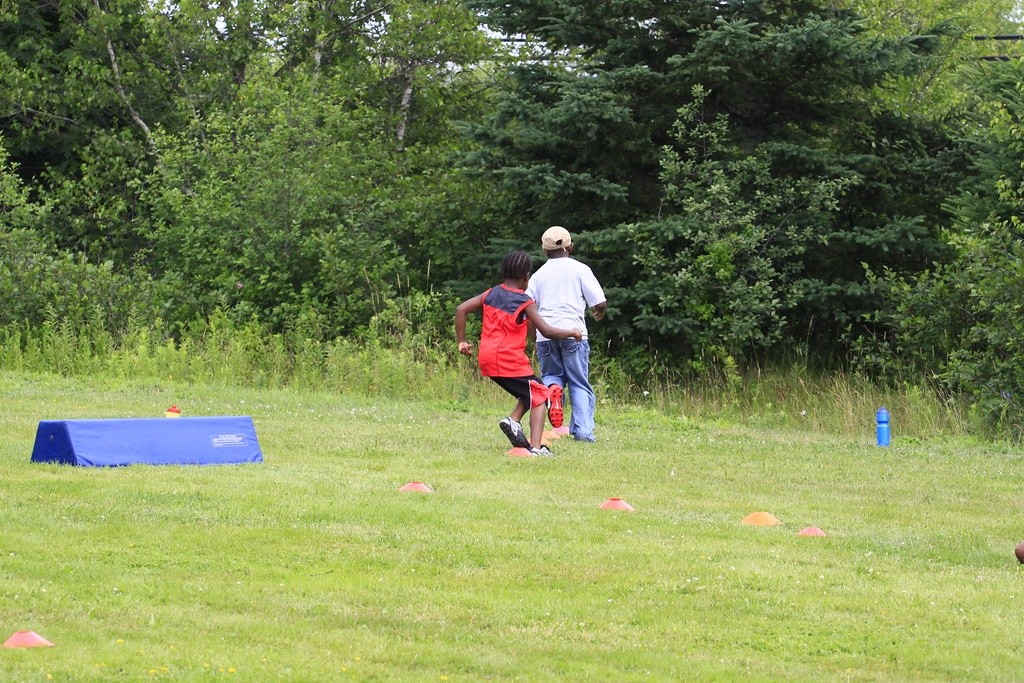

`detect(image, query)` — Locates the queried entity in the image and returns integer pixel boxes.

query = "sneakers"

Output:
[548,384,564,428]
[498,416,530,450]
[528,447,556,459]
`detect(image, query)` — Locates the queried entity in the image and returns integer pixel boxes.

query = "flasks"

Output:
[877,408,890,446]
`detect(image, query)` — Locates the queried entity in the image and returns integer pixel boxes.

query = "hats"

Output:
[540,225,572,251]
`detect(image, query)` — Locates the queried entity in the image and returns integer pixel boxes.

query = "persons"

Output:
[524,226,608,443]
[455,252,582,459]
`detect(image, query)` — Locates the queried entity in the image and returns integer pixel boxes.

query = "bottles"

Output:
[164,405,180,418]
